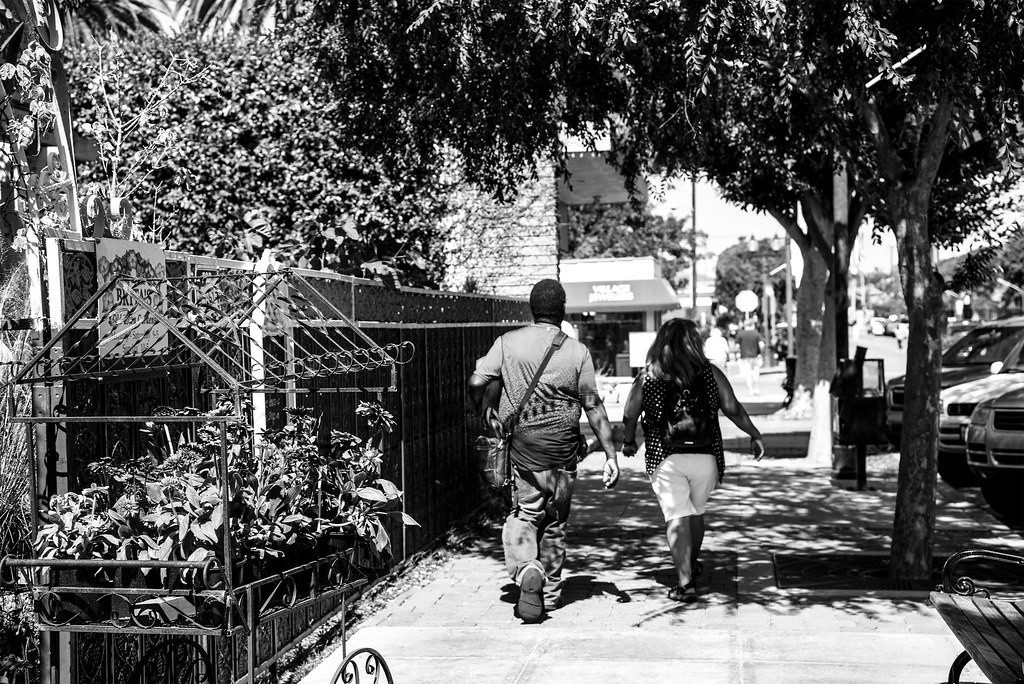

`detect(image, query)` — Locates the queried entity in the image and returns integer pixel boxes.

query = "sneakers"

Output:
[517,567,545,624]
[542,595,559,610]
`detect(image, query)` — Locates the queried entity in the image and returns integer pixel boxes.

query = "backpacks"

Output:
[660,374,714,448]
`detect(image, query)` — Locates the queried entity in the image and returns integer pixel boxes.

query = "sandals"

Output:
[691,560,704,578]
[668,582,697,603]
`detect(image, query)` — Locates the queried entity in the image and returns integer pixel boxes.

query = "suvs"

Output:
[963,384,1024,516]
[937,334,1024,490]
[887,315,1024,447]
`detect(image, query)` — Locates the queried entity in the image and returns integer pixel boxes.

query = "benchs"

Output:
[930,549,1024,684]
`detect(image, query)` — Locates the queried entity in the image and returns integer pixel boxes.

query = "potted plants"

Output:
[33,393,421,631]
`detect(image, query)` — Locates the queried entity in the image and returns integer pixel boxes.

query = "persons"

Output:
[734,318,765,398]
[698,314,726,339]
[620,317,766,601]
[701,327,729,374]
[465,278,620,623]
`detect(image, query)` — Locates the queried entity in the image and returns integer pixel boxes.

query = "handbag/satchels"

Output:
[474,407,510,489]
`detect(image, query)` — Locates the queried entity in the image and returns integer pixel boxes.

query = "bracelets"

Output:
[623,438,636,448]
